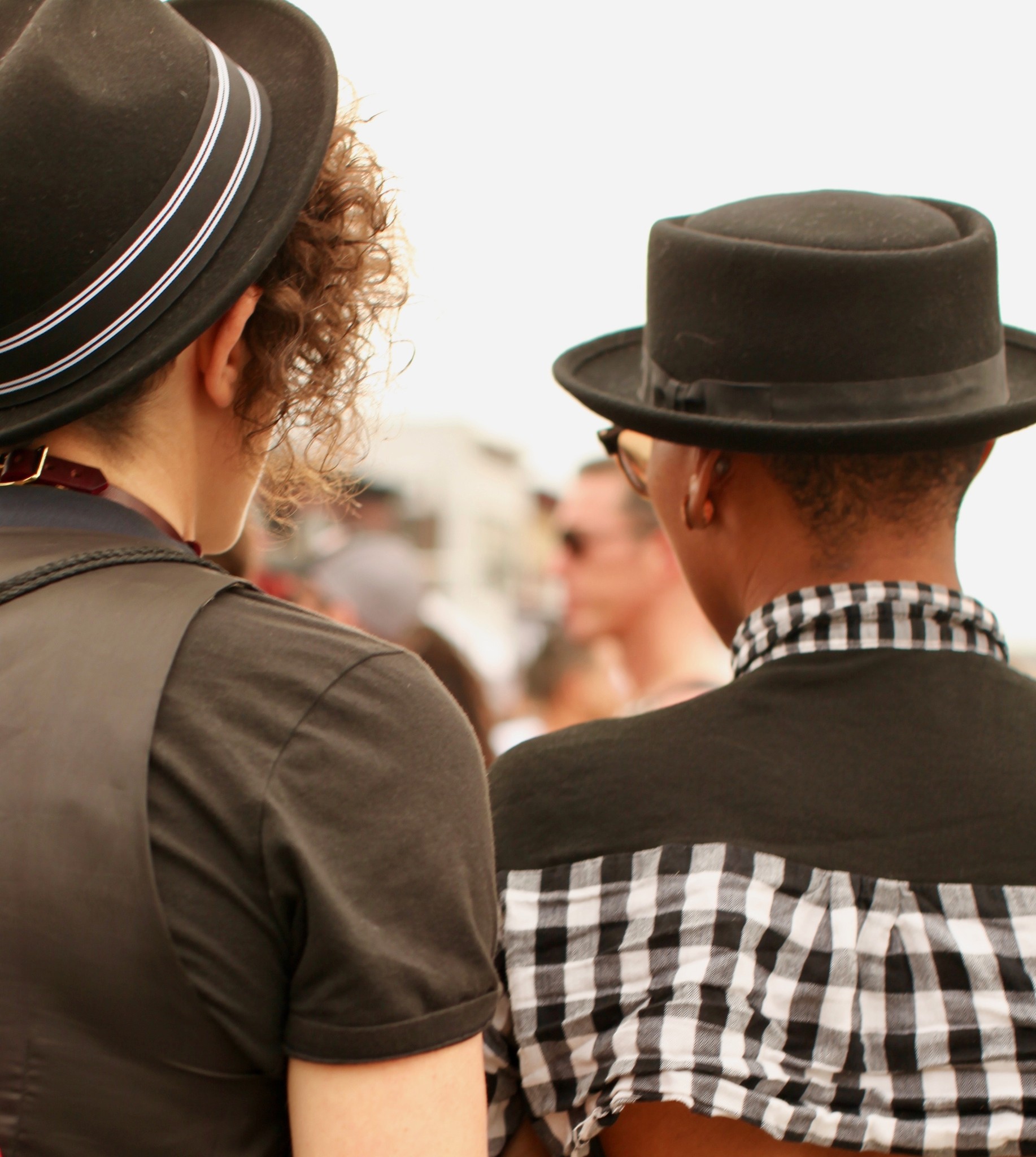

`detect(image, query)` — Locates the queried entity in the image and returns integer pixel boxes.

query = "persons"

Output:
[219,454,726,752]
[0,0,502,1157]
[488,189,1036,1157]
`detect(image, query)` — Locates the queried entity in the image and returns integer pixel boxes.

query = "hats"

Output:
[0,0,338,444]
[553,190,1036,454]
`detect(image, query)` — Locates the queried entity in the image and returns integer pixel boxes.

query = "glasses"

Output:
[557,527,595,556]
[597,424,649,497]
